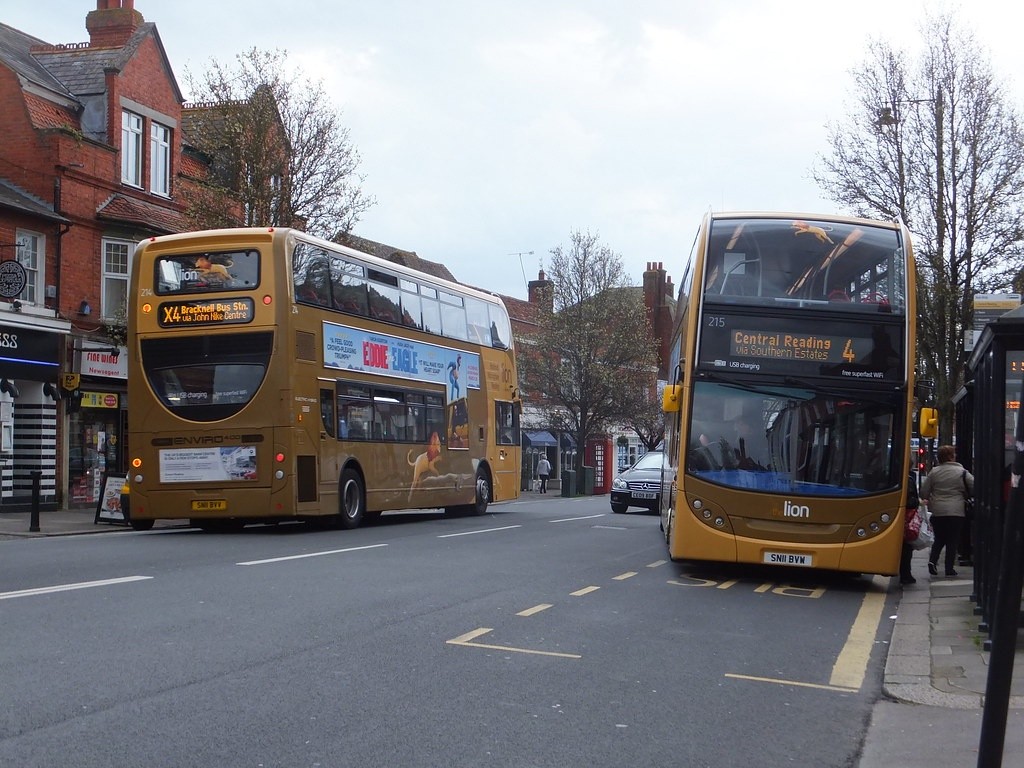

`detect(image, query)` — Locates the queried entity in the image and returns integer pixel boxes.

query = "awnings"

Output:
[522,429,558,448]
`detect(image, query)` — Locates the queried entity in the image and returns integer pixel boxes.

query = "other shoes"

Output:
[904,568,916,583]
[928,562,938,576]
[945,568,959,578]
[540,487,542,494]
[959,560,973,567]
[544,489,546,493]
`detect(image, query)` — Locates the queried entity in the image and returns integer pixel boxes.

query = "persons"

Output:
[698,413,763,473]
[903,445,980,585]
[538,454,551,493]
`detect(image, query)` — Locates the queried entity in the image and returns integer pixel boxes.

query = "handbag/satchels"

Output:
[962,470,975,518]
[910,504,935,550]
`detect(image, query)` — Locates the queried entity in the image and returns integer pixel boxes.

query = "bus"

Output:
[128,225,524,530]
[660,211,937,580]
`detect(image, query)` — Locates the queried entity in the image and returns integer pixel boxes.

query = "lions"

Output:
[790,220,835,244]
[407,431,442,504]
[191,258,234,283]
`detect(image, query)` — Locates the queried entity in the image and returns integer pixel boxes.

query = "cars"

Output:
[609,449,664,514]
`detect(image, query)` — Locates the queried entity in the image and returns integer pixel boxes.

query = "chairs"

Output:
[296,288,411,326]
[828,290,850,301]
[858,291,889,305]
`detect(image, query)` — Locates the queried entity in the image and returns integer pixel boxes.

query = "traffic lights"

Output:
[919,439,926,473]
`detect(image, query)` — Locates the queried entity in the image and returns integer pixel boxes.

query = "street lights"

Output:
[872,84,955,446]
